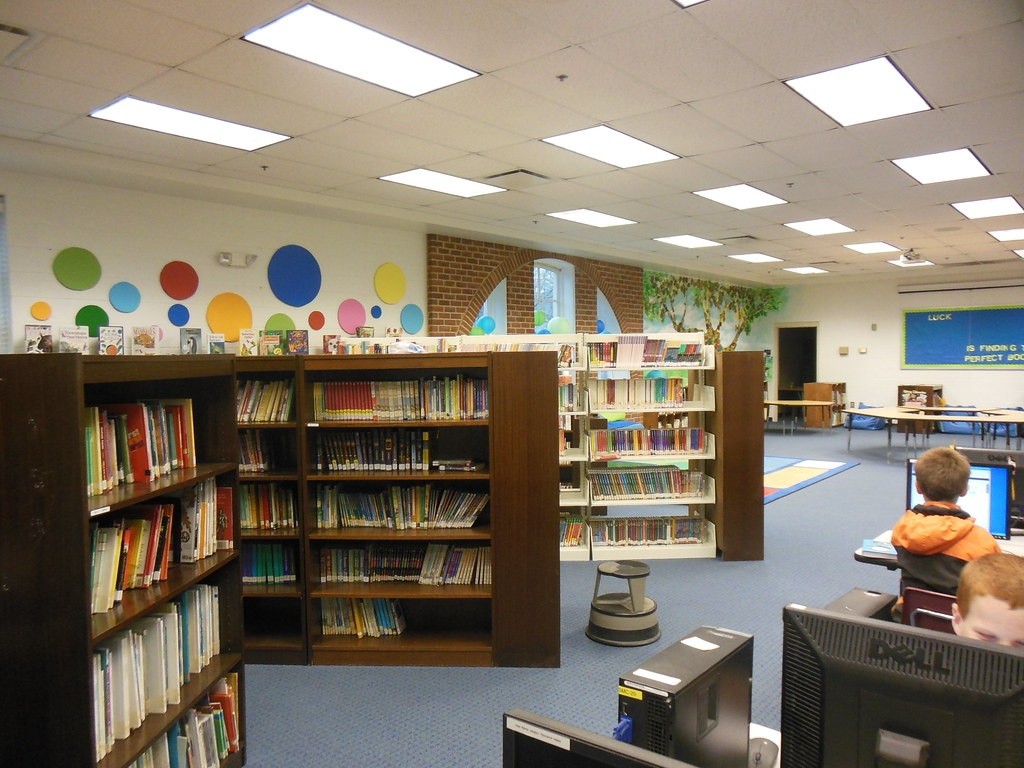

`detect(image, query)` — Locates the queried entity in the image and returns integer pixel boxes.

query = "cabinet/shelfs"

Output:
[235,356,308,665]
[804,382,846,429]
[445,331,590,561]
[0,353,248,768]
[898,384,943,434]
[584,332,716,561]
[705,351,764,562]
[300,351,561,668]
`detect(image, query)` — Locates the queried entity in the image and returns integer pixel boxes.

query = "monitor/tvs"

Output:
[782,604,1024,768]
[906,458,1012,540]
[955,447,1024,535]
[501,710,697,768]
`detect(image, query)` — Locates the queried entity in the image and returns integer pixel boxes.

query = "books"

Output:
[24,323,556,355]
[860,538,898,560]
[82,379,249,768]
[557,328,717,550]
[232,371,496,640]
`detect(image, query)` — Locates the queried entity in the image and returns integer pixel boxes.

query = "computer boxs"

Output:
[825,587,898,622]
[618,625,754,768]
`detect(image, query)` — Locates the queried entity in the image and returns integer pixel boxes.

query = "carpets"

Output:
[764,456,861,505]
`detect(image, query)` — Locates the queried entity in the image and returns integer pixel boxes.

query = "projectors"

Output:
[900,253,927,265]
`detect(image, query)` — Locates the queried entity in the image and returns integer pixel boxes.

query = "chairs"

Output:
[901,587,957,634]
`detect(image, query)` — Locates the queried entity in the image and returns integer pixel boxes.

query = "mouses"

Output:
[748,738,779,768]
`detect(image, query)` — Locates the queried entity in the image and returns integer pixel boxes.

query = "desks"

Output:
[854,528,1024,571]
[764,400,835,436]
[779,387,803,399]
[838,406,1024,467]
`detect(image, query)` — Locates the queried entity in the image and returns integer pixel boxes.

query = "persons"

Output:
[950,552,1024,656]
[890,445,1004,625]
